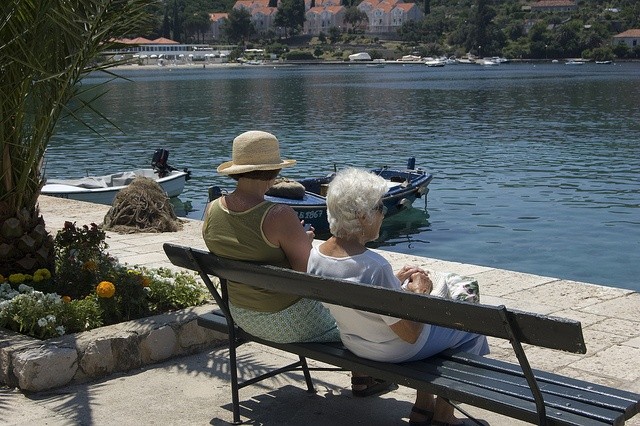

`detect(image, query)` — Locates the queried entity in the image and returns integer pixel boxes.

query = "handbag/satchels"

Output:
[401,268,480,303]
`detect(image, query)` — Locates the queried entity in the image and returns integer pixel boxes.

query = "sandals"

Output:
[351,377,392,397]
[430,419,468,426]
[409,406,434,426]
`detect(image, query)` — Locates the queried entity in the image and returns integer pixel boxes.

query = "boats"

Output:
[596,60,612,63]
[208,157,433,228]
[425,61,446,66]
[367,64,384,68]
[456,55,478,63]
[565,60,585,64]
[476,58,498,65]
[38,168,188,204]
[492,56,510,62]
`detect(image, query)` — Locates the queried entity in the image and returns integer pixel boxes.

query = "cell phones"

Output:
[303,223,311,232]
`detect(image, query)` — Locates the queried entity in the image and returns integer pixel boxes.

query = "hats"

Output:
[217,130,297,176]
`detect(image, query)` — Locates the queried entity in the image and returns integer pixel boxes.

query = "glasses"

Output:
[372,204,387,215]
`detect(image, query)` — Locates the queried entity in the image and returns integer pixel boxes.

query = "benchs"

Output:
[163,243,640,426]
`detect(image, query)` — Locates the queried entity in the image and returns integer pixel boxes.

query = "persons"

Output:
[307,166,491,425]
[202,130,393,398]
